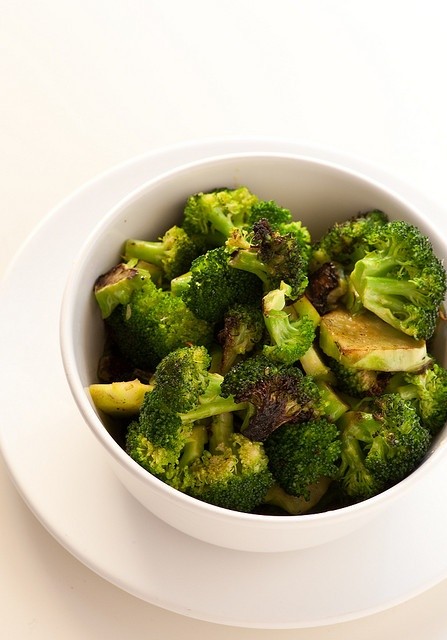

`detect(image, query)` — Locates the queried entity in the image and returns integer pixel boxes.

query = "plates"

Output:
[0,136,446,629]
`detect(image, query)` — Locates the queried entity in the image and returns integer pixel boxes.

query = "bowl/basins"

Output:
[61,152,446,554]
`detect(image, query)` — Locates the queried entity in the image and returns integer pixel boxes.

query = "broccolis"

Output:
[87,186,446,513]
